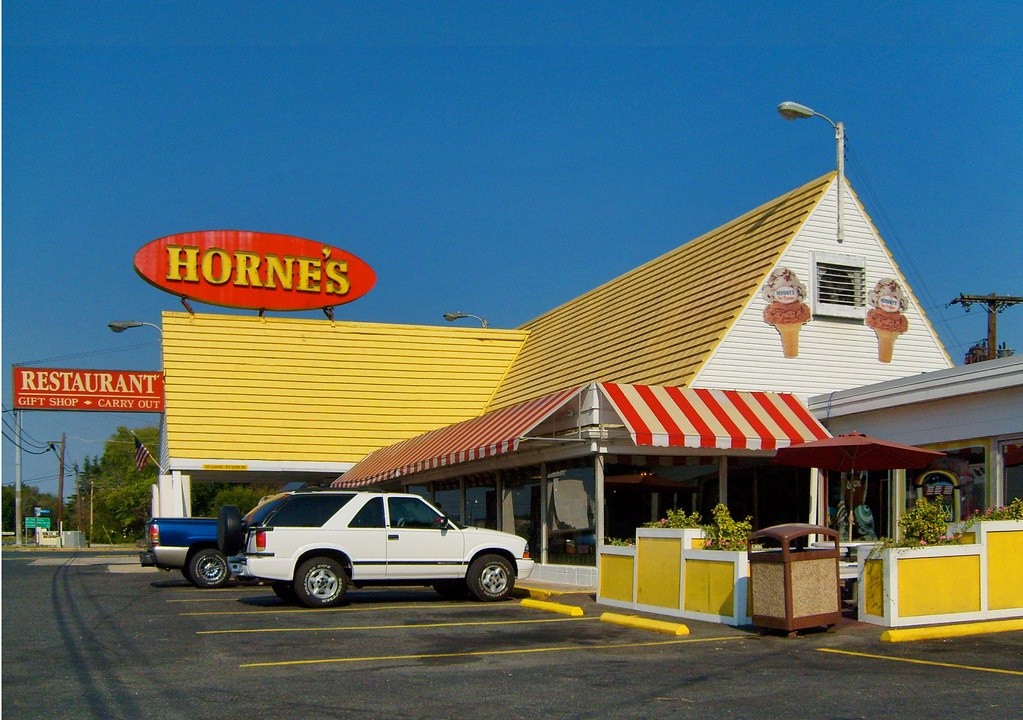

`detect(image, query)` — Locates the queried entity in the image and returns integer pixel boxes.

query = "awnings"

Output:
[329,375,591,489]
[595,381,833,451]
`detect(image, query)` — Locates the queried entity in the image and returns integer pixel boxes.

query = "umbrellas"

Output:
[776,430,946,599]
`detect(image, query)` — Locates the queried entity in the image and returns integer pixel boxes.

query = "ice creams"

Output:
[762,267,812,360]
[866,277,909,363]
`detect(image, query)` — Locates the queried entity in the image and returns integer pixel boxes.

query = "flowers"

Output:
[656,518,670,527]
[918,503,1012,546]
[704,539,746,550]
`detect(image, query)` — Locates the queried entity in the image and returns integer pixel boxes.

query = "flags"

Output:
[134,436,149,473]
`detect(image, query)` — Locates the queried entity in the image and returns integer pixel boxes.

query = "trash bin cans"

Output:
[746,524,843,641]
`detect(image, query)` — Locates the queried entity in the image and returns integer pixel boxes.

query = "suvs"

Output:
[217,492,535,608]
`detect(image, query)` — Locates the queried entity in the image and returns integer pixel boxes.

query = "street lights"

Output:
[108,322,162,336]
[443,310,488,329]
[777,101,845,242]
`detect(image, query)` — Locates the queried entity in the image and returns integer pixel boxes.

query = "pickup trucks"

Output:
[139,517,260,588]
[530,528,596,566]
